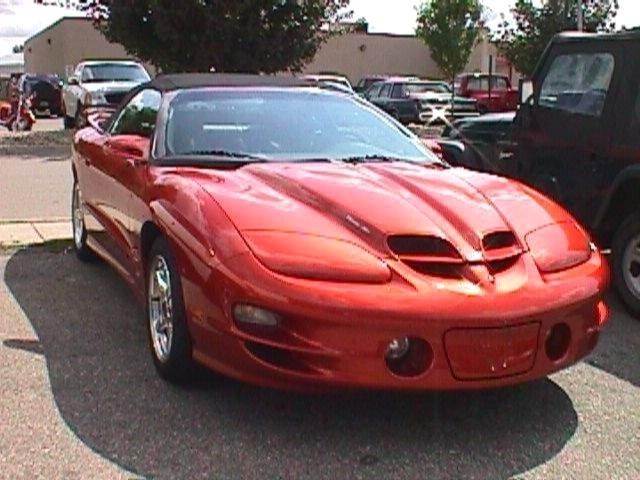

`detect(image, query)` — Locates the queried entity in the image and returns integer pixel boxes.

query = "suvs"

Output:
[438,31,640,317]
[59,58,152,130]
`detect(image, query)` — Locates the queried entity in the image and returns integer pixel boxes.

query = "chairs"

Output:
[173,111,204,154]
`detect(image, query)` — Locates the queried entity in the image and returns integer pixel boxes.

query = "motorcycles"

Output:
[0,77,36,130]
